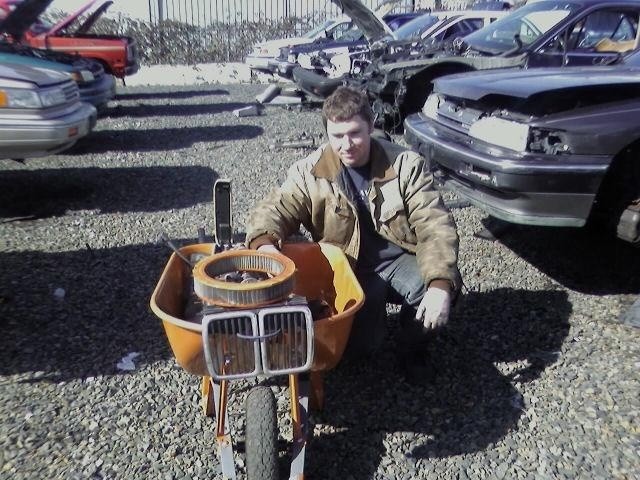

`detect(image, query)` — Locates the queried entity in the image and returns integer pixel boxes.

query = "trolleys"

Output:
[149,228,367,480]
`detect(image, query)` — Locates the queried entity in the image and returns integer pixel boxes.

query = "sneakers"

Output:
[391,360,438,388]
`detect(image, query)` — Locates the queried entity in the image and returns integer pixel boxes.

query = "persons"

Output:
[244,85,466,369]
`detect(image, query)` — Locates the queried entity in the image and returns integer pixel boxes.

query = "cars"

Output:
[400,0,639,277]
[0,0,140,165]
[243,0,639,136]
